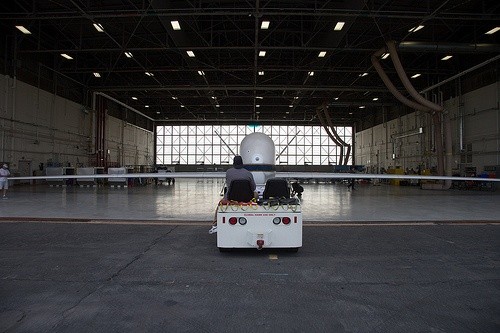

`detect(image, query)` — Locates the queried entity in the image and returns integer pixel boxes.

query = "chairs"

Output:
[227,180,254,203]
[263,179,289,200]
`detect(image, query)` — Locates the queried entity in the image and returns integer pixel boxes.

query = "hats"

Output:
[233,156,243,169]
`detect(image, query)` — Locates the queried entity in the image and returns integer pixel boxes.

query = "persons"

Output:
[0,164,10,199]
[348,168,356,189]
[208,156,259,235]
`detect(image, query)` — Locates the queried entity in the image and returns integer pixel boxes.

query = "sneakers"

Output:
[208,225,217,234]
